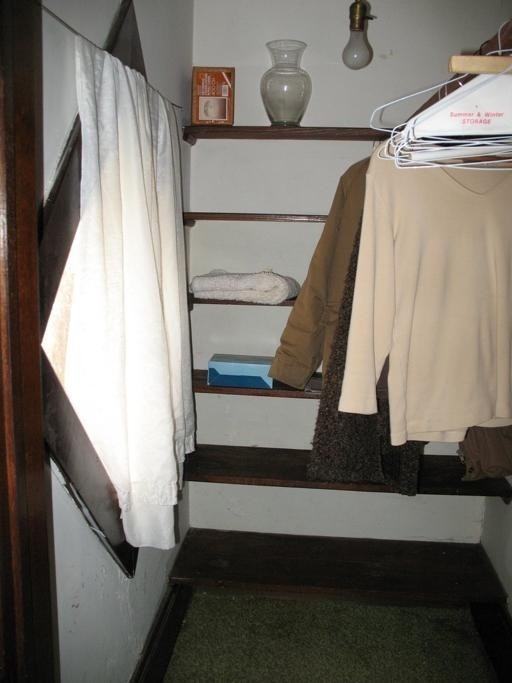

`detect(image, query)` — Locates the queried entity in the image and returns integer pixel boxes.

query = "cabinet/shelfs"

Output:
[168,125,512,610]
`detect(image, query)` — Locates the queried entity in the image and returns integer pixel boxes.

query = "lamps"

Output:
[342,0,371,70]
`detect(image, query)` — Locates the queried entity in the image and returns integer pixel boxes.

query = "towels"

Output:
[65,35,197,551]
[189,269,300,305]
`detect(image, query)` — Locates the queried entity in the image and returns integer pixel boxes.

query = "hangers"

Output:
[369,19,512,171]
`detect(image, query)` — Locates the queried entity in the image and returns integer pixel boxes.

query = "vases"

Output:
[260,39,312,127]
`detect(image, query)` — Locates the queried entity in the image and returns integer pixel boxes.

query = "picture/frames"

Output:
[191,66,235,126]
